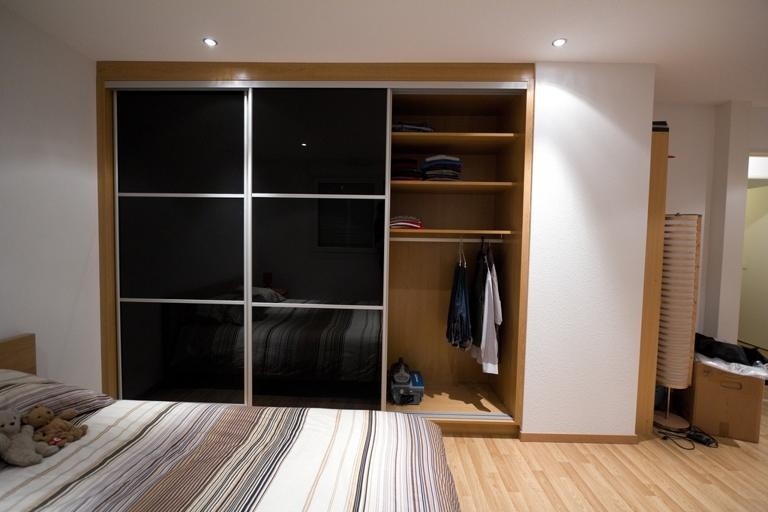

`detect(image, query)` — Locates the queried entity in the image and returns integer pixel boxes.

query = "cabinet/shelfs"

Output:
[97,60,535,441]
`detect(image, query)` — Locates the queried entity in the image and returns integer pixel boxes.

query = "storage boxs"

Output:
[687,357,766,444]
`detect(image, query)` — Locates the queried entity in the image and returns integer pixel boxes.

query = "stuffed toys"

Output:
[0,407,60,469]
[21,402,90,448]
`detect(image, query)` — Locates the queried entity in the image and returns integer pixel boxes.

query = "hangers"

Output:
[454,236,496,277]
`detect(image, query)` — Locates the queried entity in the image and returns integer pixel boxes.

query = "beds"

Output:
[1,332,468,512]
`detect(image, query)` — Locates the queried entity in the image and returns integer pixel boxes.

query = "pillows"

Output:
[1,366,114,468]
